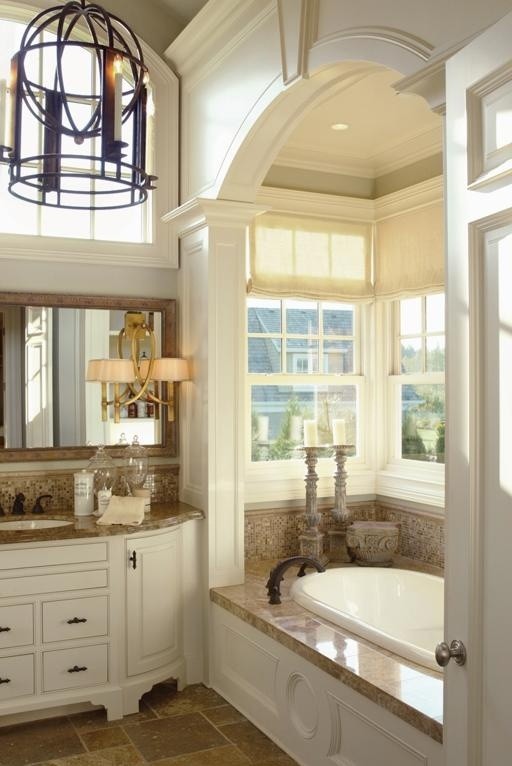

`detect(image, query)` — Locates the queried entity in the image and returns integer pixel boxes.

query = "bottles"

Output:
[128,395,154,419]
[72,435,151,517]
[114,433,131,447]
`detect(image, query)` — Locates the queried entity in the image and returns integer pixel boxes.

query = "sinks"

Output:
[0,514,78,531]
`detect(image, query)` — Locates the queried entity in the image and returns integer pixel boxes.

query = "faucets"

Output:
[11,493,26,515]
[269,555,325,604]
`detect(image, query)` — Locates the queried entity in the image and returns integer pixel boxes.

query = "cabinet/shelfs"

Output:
[123,526,188,716]
[1,539,120,722]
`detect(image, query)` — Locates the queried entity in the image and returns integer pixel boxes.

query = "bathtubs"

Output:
[289,566,445,674]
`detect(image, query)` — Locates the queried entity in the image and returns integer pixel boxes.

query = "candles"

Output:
[303,418,347,447]
[256,414,302,442]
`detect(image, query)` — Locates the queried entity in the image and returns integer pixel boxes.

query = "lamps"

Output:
[86,328,162,420]
[94,311,192,423]
[0,0,158,210]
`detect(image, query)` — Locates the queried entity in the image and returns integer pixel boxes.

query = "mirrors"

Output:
[0,291,179,464]
